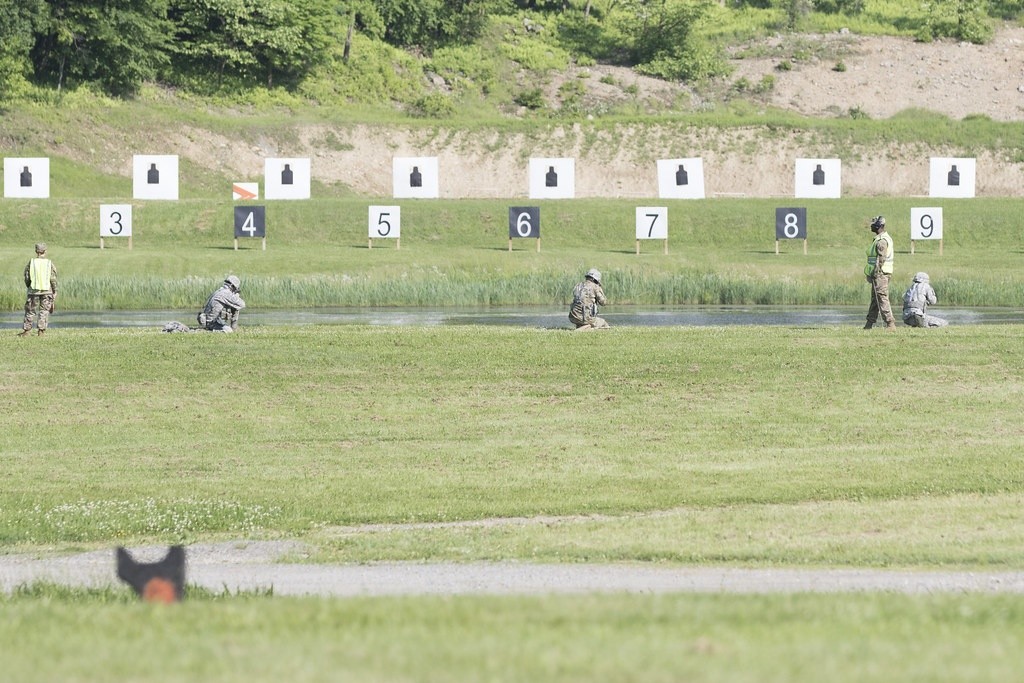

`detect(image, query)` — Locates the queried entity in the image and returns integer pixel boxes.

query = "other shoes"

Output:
[886,323,895,329]
[18,331,31,337]
[38,331,43,336]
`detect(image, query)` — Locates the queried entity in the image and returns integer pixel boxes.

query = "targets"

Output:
[265,159,310,201]
[657,159,704,199]
[394,157,439,198]
[529,159,575,198]
[929,157,976,198]
[4,156,51,198]
[133,156,179,199]
[794,159,842,199]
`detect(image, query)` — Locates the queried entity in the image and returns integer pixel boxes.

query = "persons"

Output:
[902,272,949,328]
[17,243,57,336]
[204,275,246,331]
[568,269,608,331]
[863,216,896,329]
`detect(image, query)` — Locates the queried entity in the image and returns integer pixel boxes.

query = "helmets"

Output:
[585,269,602,286]
[871,216,885,225]
[35,243,46,252]
[225,275,240,290]
[913,271,930,284]
